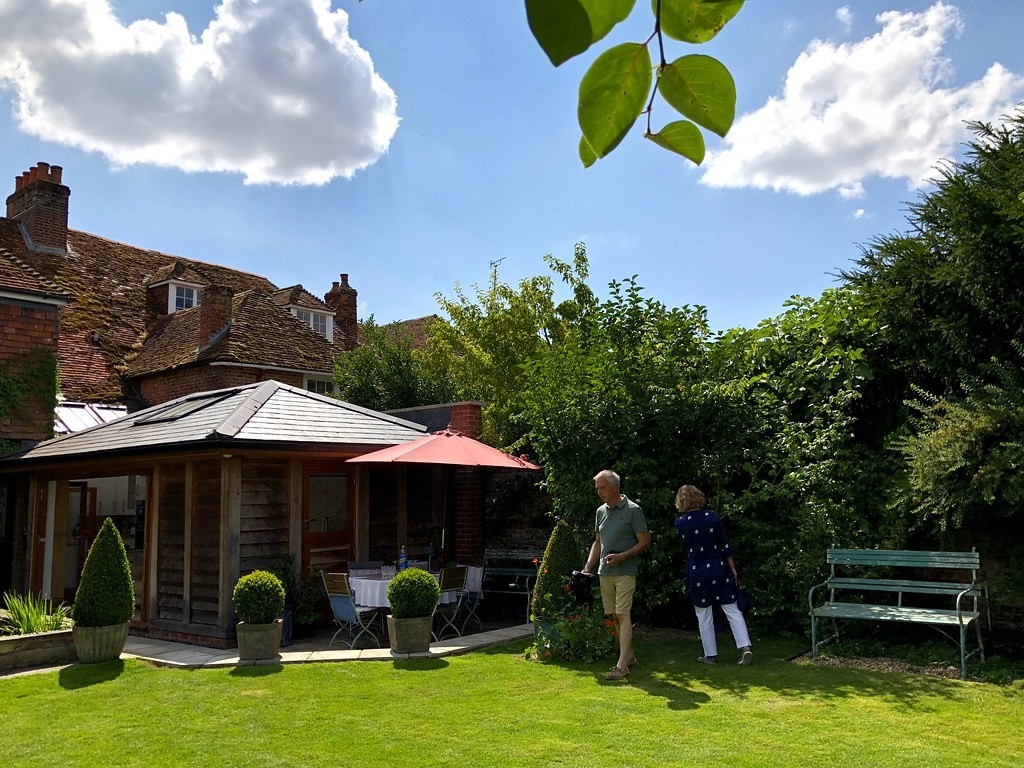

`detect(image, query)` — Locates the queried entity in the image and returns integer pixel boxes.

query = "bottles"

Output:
[400,545,406,554]
[408,560,413,567]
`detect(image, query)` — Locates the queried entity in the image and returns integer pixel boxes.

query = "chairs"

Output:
[432,566,468,641]
[465,562,488,635]
[319,569,382,648]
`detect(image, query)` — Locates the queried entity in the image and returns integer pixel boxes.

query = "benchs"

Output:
[809,544,990,680]
[480,549,545,625]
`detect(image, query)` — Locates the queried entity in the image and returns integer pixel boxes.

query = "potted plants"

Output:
[232,570,287,660]
[386,568,441,652]
[531,519,591,641]
[71,517,135,664]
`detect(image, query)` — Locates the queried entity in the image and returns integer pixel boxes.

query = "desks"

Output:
[350,574,457,610]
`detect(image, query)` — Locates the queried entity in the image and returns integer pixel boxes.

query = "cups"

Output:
[389,567,394,580]
[381,566,389,580]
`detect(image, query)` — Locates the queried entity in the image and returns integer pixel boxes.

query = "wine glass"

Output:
[413,560,418,567]
[392,561,398,568]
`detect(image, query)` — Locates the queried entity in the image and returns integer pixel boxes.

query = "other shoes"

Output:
[609,656,637,671]
[605,666,630,679]
[737,646,752,666]
[698,654,718,664]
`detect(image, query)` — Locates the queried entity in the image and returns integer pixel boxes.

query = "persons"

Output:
[583,471,649,679]
[675,486,752,665]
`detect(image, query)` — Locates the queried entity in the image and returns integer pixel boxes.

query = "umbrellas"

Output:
[345,422,541,568]
